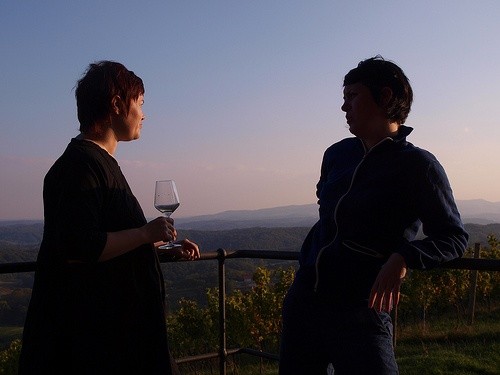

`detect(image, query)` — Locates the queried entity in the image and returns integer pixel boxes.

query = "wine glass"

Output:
[154,179,182,249]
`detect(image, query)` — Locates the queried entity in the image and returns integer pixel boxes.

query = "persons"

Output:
[278,56,470,375]
[18,59,200,375]
